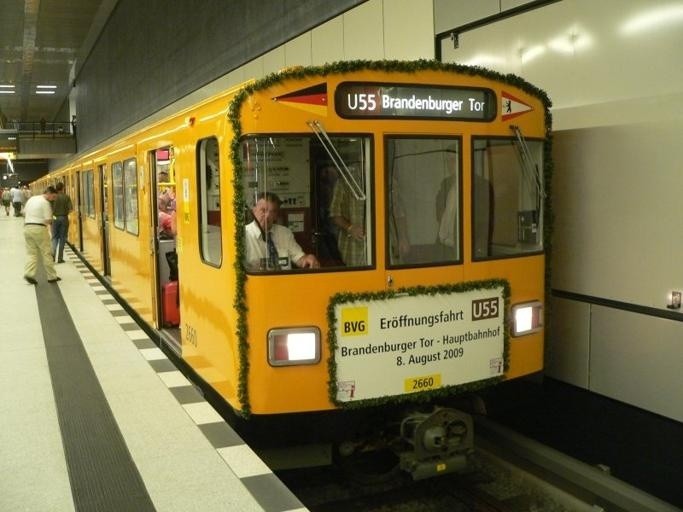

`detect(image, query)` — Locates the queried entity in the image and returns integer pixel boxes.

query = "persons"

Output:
[242,191,324,271]
[326,158,413,268]
[434,144,495,262]
[48,181,73,264]
[20,185,62,286]
[98,168,176,243]
[0,185,31,217]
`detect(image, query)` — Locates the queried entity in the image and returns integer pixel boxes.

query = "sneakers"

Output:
[24,276,39,285]
[53,258,65,264]
[46,277,62,283]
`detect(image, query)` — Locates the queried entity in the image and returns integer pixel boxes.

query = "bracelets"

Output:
[345,223,353,230]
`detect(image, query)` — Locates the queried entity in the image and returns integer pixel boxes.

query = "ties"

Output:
[267,231,281,273]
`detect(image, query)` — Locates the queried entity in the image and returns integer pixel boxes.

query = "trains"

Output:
[18,60,553,499]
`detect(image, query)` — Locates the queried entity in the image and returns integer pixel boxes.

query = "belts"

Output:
[22,222,47,226]
